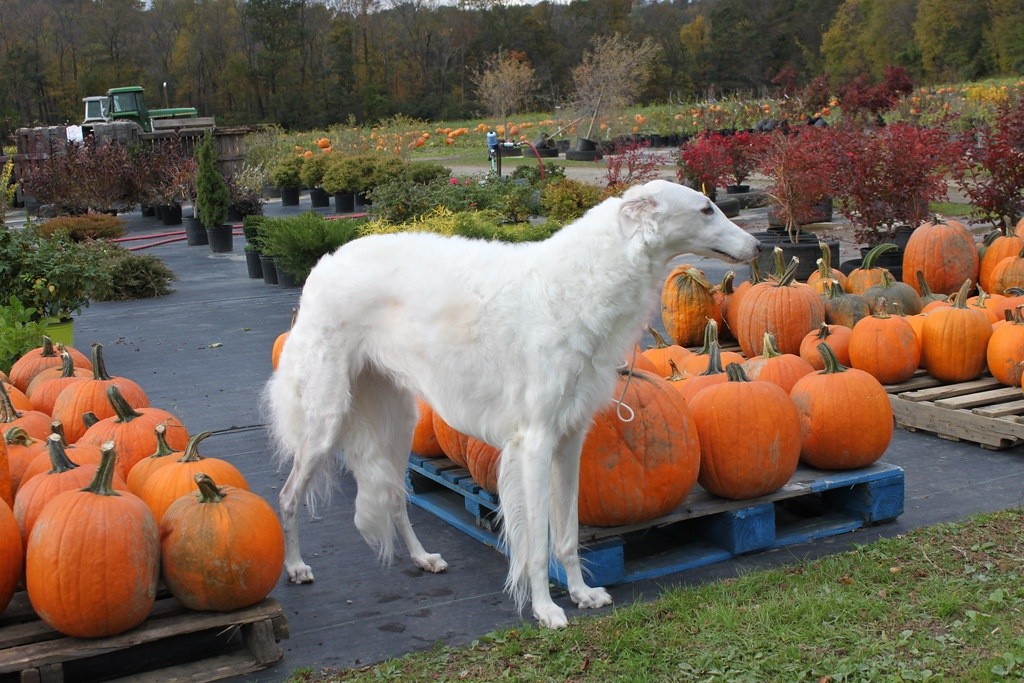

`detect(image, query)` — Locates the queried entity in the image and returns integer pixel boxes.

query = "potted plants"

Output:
[355,120,376,206]
[0,226,180,347]
[161,158,182,226]
[181,127,224,246]
[131,143,154,216]
[751,64,1024,282]
[20,138,90,217]
[529,28,749,217]
[243,214,270,278]
[270,128,299,206]
[240,123,288,198]
[255,218,279,285]
[226,160,270,222]
[65,135,134,216]
[321,113,368,212]
[147,144,178,220]
[270,221,299,289]
[464,42,544,148]
[194,143,233,252]
[282,128,330,208]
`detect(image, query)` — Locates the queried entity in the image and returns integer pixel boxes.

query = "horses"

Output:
[261,179,762,629]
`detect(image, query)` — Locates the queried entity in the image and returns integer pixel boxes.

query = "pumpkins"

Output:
[295,81,1024,160]
[269,214,1024,527]
[0,335,286,637]
